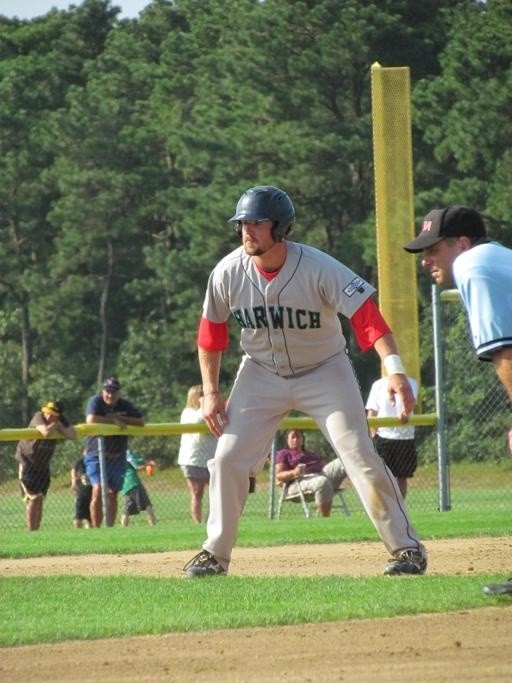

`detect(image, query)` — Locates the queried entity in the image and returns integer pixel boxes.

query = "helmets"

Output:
[228,184,295,241]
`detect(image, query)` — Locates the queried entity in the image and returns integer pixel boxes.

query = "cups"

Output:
[298,462,307,480]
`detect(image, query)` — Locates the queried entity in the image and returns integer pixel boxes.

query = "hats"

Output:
[403,204,487,254]
[102,377,119,391]
[40,400,64,416]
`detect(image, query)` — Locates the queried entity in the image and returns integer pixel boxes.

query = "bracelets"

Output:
[383,354,405,375]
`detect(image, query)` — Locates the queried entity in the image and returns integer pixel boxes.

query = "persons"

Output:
[86,377,145,529]
[121,441,159,529]
[71,448,99,532]
[366,375,418,500]
[405,206,512,601]
[181,187,434,582]
[177,383,227,524]
[16,399,76,531]
[278,427,351,519]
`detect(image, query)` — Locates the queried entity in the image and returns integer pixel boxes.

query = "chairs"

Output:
[273,467,352,520]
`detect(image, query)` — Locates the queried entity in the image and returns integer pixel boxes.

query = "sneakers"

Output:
[187,550,226,578]
[381,550,428,577]
